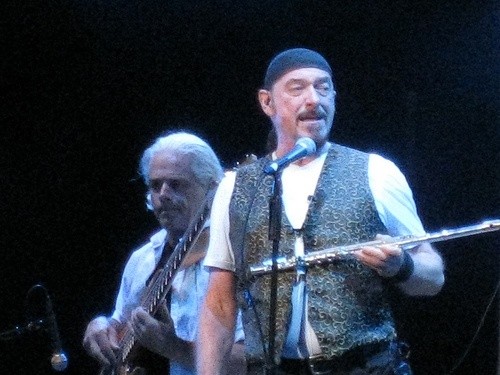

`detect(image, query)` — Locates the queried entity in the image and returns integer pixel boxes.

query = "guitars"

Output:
[98,153,257,375]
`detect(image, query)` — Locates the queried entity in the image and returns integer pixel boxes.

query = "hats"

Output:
[263,47,332,90]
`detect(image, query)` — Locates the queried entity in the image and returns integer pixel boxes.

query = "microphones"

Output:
[46,296,68,371]
[264,136,316,175]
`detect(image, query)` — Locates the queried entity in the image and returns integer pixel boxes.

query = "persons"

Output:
[193,48,446,375]
[82,130,249,375]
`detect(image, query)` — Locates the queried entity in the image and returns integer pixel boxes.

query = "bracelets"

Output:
[386,248,414,285]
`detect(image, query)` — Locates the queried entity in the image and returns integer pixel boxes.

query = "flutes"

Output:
[250,219,500,276]
